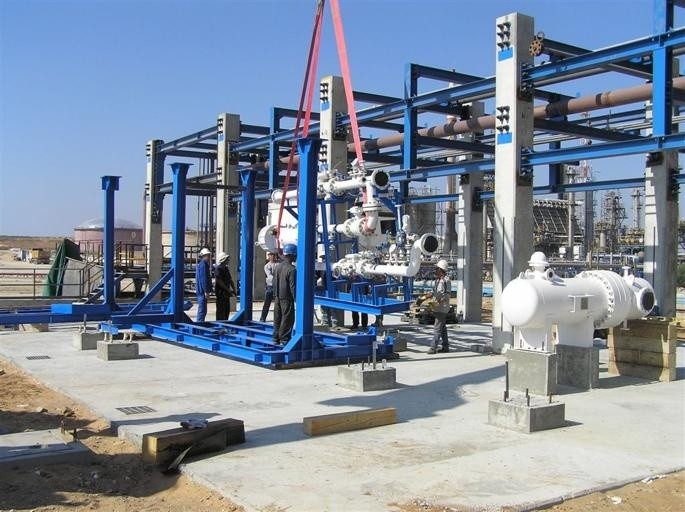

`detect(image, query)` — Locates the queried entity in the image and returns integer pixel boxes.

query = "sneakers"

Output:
[428,348,449,354]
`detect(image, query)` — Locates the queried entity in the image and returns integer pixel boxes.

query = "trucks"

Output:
[27,248,51,264]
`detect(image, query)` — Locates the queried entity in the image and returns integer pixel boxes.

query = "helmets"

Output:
[434,260,448,273]
[283,244,297,256]
[217,252,229,263]
[199,248,211,256]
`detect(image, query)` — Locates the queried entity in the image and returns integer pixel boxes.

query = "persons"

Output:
[260,251,281,322]
[349,274,368,330]
[272,244,297,344]
[317,255,327,316]
[196,248,213,321]
[214,252,237,320]
[428,260,451,354]
